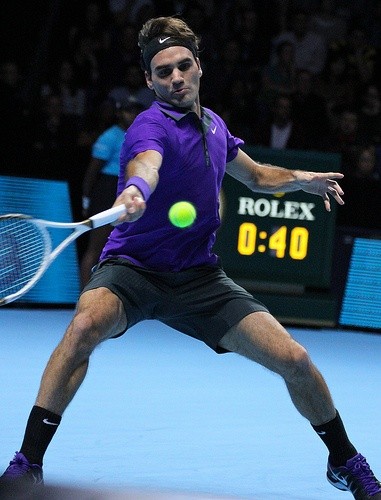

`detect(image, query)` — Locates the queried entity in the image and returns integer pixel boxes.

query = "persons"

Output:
[0,0,381,238]
[0,15,381,500]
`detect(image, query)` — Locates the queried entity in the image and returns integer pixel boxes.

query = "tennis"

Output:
[169,202,197,229]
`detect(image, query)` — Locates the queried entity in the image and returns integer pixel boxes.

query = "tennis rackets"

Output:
[0,203,127,310]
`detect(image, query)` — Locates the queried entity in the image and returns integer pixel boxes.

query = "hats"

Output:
[115,95,146,111]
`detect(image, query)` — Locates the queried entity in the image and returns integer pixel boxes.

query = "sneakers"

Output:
[326,452,381,500]
[1,450,45,483]
